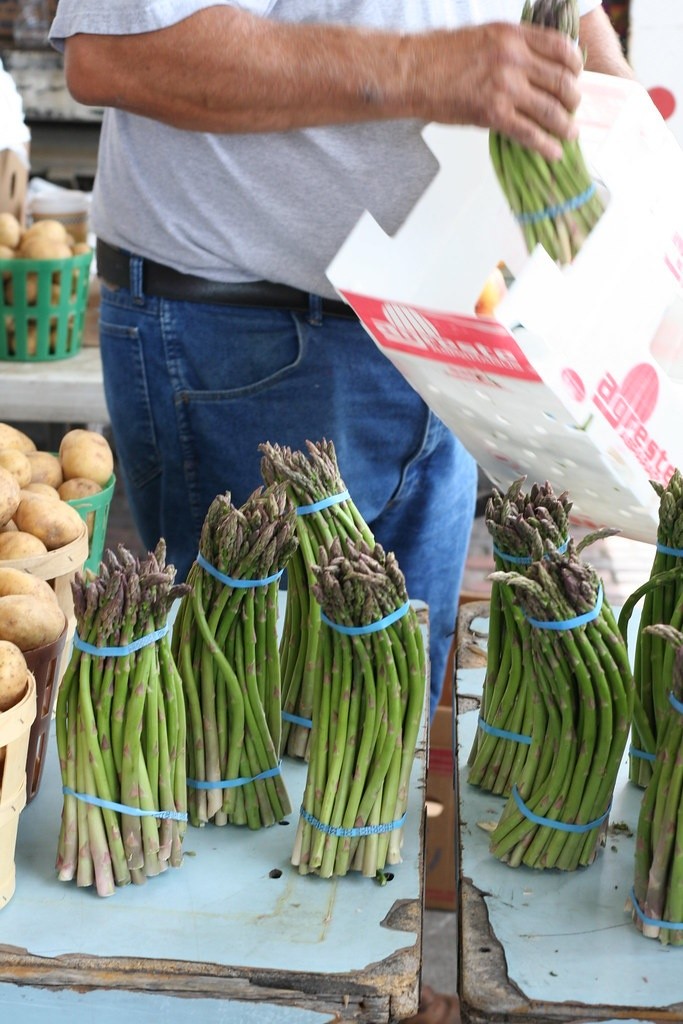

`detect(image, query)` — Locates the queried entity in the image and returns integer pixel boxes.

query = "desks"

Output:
[452,600,683,1024]
[0,599,431,1024]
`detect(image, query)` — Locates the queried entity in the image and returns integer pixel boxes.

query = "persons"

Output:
[45,0,641,741]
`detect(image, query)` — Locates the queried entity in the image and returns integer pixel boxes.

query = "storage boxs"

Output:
[323,70,683,543]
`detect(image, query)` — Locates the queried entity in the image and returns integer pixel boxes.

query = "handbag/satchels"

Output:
[328,69,683,546]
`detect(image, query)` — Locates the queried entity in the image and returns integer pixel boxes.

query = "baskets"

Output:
[95,236,362,320]
[24,612,70,805]
[0,519,91,642]
[1,667,36,911]
[52,449,117,577]
[0,248,90,361]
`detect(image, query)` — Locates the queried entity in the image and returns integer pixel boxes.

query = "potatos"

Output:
[2,416,116,759]
[2,204,91,362]
